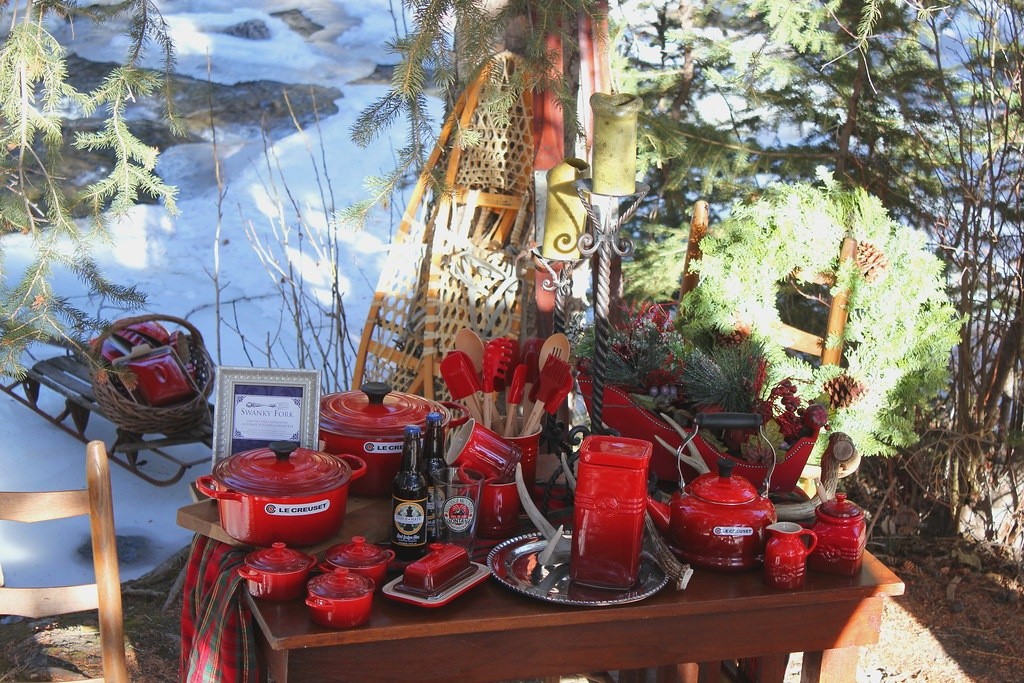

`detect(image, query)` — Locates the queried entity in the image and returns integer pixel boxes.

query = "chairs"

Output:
[0,440,129,682]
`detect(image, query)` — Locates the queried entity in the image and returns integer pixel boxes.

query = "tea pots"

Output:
[646,412,777,573]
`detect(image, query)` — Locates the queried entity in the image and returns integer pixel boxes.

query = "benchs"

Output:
[0,356,215,487]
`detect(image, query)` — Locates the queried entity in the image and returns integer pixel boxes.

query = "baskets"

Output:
[92,314,215,434]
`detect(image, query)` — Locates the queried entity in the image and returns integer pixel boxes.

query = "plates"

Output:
[91,317,196,407]
[382,560,491,609]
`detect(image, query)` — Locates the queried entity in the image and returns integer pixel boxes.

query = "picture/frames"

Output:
[212,366,321,474]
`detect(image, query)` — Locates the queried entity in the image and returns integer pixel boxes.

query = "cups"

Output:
[445,418,524,485]
[486,416,543,486]
[456,479,520,540]
[763,521,817,589]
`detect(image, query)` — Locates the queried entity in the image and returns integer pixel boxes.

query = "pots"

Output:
[194,441,368,545]
[318,381,471,496]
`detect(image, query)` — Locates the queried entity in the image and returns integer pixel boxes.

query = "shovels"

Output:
[454,328,485,375]
[522,334,574,438]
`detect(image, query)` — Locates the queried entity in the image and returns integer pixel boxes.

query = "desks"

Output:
[237,548,908,683]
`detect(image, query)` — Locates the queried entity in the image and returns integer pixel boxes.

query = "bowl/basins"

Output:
[237,542,317,600]
[317,535,395,587]
[306,567,375,630]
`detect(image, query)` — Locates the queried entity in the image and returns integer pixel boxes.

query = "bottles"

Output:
[388,411,448,560]
[808,492,867,577]
